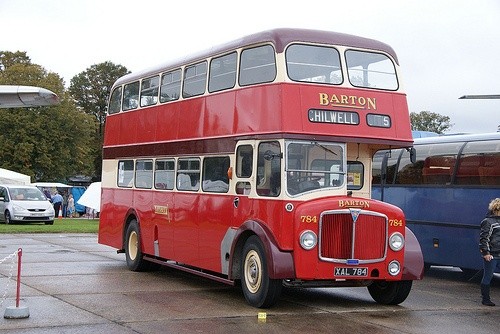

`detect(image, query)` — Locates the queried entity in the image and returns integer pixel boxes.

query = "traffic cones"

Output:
[57,206,63,220]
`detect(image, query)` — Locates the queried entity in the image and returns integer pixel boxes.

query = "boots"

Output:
[480,284,495,306]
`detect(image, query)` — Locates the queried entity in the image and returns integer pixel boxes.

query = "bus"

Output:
[98,27,424,306]
[336,130,500,275]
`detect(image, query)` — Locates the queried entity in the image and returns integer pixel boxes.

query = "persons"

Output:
[67,194,75,218]
[479,198,500,306]
[43,189,53,203]
[50,191,63,218]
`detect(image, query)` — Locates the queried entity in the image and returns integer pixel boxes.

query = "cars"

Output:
[0,184,55,225]
